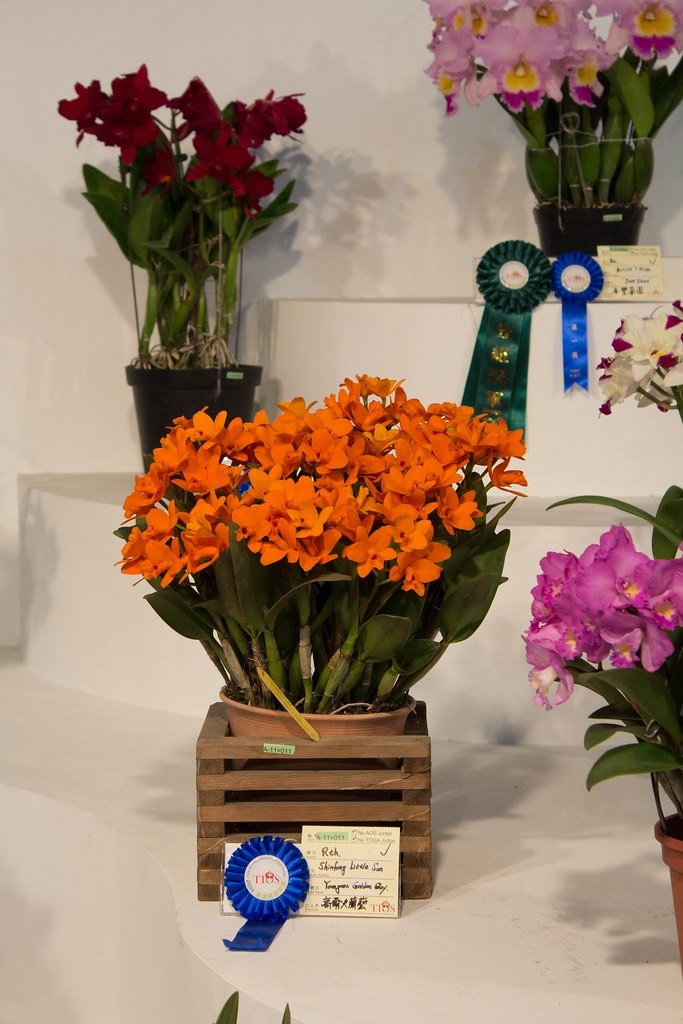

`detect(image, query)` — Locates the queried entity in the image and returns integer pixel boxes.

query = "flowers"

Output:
[520,303,683,838]
[424,0,683,205]
[115,376,527,743]
[58,64,308,367]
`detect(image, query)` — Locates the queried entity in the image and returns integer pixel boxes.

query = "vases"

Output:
[124,363,264,475]
[218,683,415,796]
[654,814,683,969]
[531,202,647,257]
